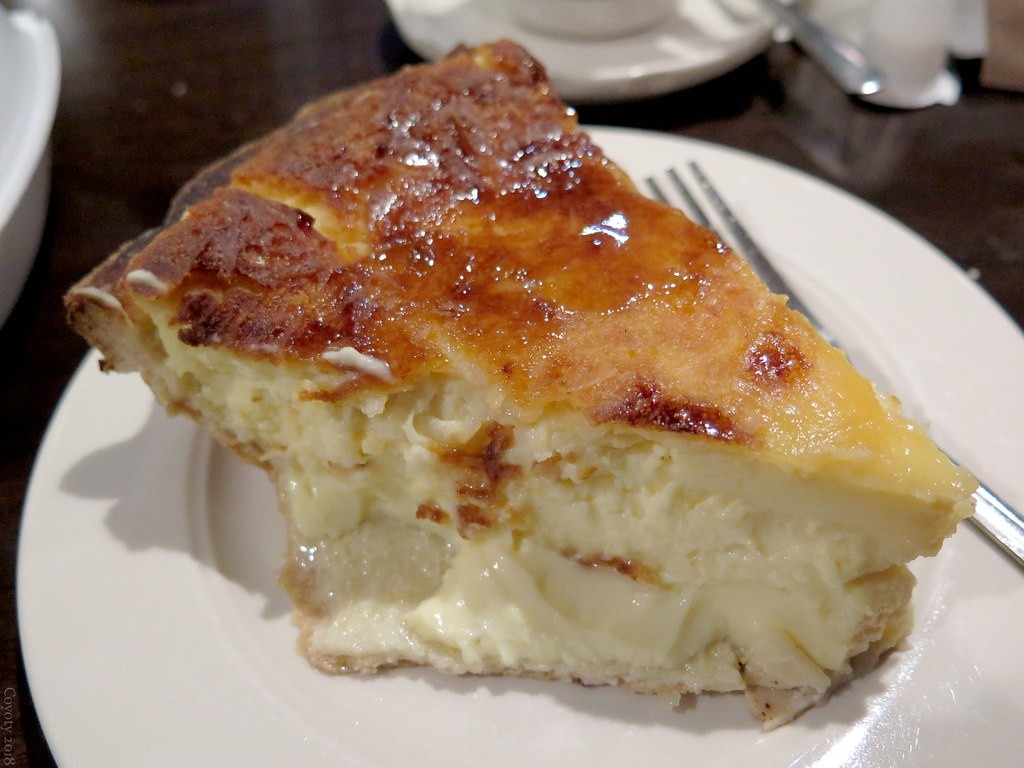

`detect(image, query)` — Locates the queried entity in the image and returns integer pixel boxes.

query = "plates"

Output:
[16,129,1024,768]
[387,0,797,101]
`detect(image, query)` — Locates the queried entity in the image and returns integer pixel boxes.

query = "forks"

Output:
[646,162,1024,566]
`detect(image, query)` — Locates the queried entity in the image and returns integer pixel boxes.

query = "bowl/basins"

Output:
[0,1,60,323]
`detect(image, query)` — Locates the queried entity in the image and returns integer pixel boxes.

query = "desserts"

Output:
[63,39,978,732]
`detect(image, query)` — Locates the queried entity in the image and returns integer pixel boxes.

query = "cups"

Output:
[510,0,676,41]
